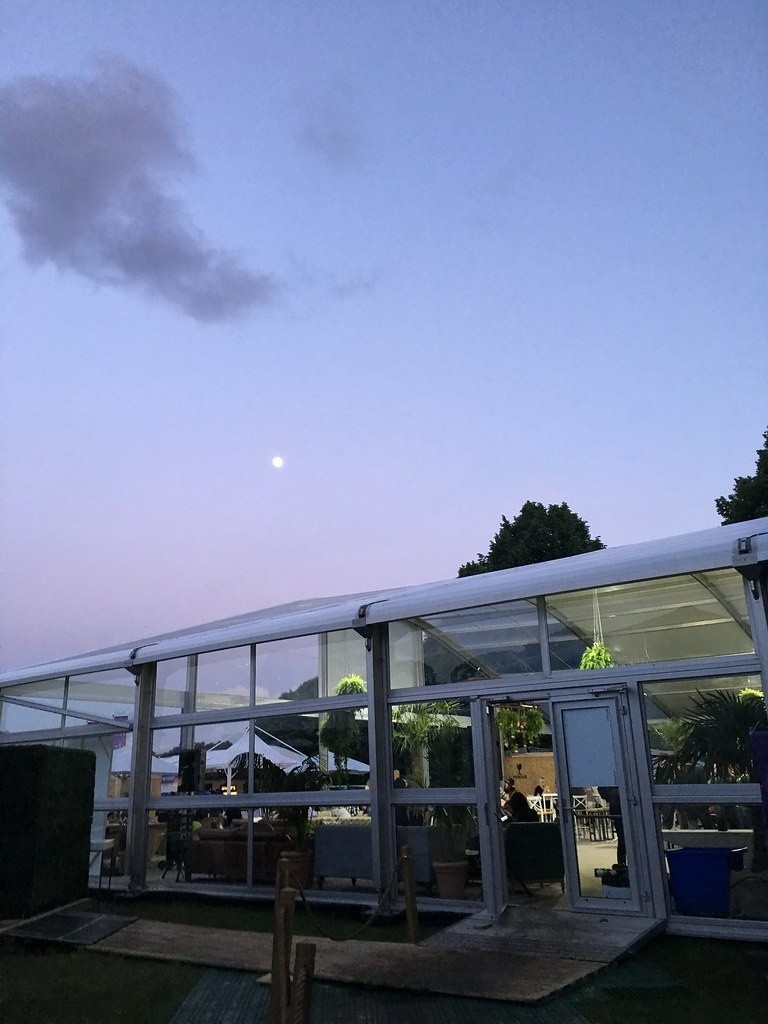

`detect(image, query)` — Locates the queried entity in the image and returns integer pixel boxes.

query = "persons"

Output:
[503,778,532,822]
[394,770,406,789]
[572,786,602,827]
[598,786,627,869]
[534,777,559,822]
[656,756,734,830]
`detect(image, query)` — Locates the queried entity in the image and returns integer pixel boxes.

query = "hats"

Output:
[502,778,515,787]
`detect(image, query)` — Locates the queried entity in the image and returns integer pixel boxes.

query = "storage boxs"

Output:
[601,885,631,899]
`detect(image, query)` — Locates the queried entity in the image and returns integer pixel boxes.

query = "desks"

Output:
[585,807,615,841]
[90,839,116,888]
[538,809,553,823]
[661,829,754,869]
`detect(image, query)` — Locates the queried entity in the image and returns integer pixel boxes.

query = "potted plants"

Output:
[275,806,314,889]
[102,792,129,876]
[427,805,477,899]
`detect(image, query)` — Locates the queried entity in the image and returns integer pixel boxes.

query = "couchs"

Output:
[502,821,567,895]
[183,829,292,885]
[314,824,446,897]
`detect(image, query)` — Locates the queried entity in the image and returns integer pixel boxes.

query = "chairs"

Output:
[664,848,733,919]
[162,832,182,882]
[527,793,589,840]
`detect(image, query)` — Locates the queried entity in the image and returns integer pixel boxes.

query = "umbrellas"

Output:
[110,730,370,796]
